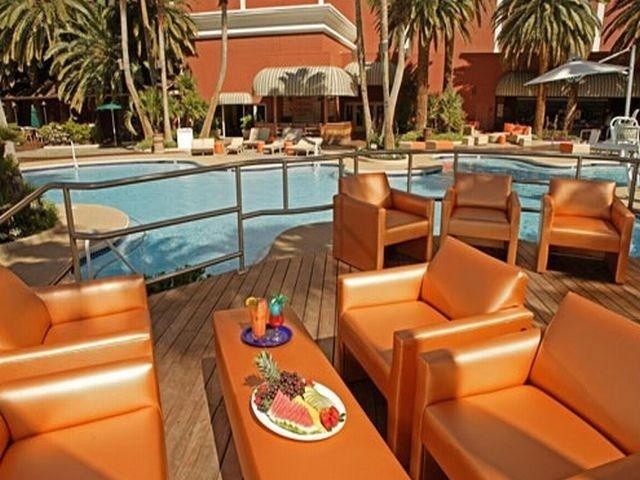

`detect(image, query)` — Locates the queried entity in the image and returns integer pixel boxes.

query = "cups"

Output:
[246,296,268,343]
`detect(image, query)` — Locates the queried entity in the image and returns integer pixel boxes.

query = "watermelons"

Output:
[266,389,319,436]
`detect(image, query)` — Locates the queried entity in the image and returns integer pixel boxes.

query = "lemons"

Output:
[245,296,258,306]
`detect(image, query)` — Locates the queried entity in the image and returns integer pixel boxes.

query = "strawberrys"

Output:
[320,406,345,431]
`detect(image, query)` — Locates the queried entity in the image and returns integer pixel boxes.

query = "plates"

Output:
[250,380,349,442]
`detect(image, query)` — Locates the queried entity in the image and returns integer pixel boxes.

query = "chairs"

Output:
[191,113,640,159]
[0,357,169,480]
[440,170,528,261]
[328,169,437,270]
[1,260,158,384]
[536,176,634,283]
[334,234,535,473]
[407,287,638,479]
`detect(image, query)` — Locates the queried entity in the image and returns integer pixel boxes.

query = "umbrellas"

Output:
[30,104,40,128]
[95,101,123,146]
[522,56,632,86]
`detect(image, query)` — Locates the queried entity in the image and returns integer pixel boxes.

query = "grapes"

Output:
[254,371,305,412]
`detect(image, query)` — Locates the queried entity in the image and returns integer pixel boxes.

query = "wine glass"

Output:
[271,308,286,345]
